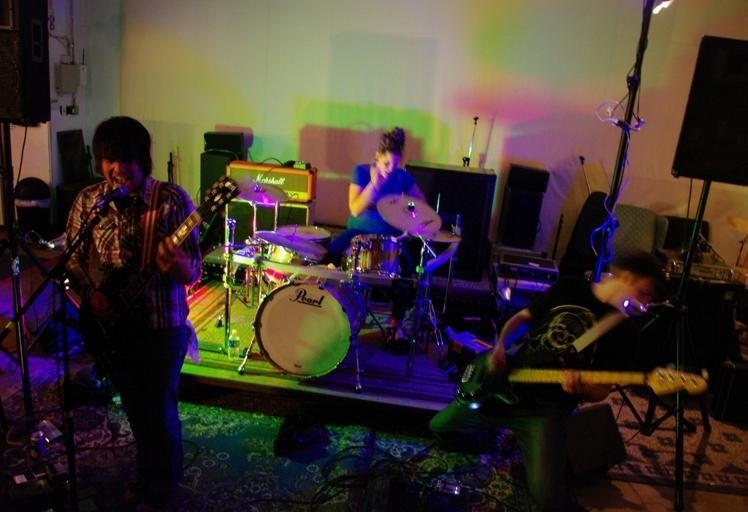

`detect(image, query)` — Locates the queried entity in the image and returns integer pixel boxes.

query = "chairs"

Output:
[611,202,668,269]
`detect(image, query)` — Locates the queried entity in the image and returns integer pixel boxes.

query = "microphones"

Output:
[94,186,129,207]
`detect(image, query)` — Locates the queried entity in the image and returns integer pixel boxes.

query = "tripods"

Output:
[0,132,56,429]
[391,246,448,381]
[606,187,710,511]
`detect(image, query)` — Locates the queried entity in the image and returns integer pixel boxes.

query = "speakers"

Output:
[492,188,545,255]
[201,151,238,207]
[0,0,50,128]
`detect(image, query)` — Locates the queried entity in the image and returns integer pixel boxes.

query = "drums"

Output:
[251,230,329,284]
[276,225,332,250]
[344,235,402,285]
[255,265,365,379]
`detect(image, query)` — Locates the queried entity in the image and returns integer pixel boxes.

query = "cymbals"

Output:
[236,182,289,205]
[376,195,443,236]
[417,231,464,241]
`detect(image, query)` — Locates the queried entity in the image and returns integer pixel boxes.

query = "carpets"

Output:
[570,381,748,497]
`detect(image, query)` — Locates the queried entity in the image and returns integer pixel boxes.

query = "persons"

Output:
[330,126,427,341]
[487,253,666,511]
[65,116,203,496]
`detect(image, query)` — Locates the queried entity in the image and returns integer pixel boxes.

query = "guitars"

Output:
[458,334,707,409]
[80,175,238,361]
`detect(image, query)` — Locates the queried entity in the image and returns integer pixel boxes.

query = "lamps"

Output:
[49,13,88,114]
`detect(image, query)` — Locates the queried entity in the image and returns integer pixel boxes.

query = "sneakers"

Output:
[391,319,409,340]
